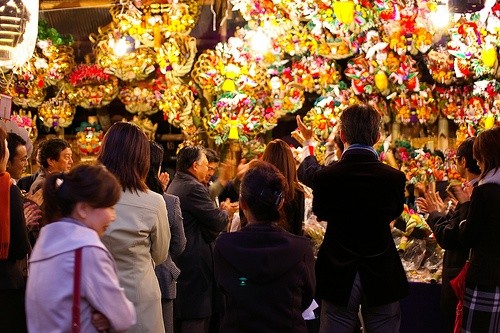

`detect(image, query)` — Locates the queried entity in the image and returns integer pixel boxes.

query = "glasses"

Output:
[453,154,461,161]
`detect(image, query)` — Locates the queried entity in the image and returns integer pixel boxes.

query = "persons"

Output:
[0,130,500,332]
